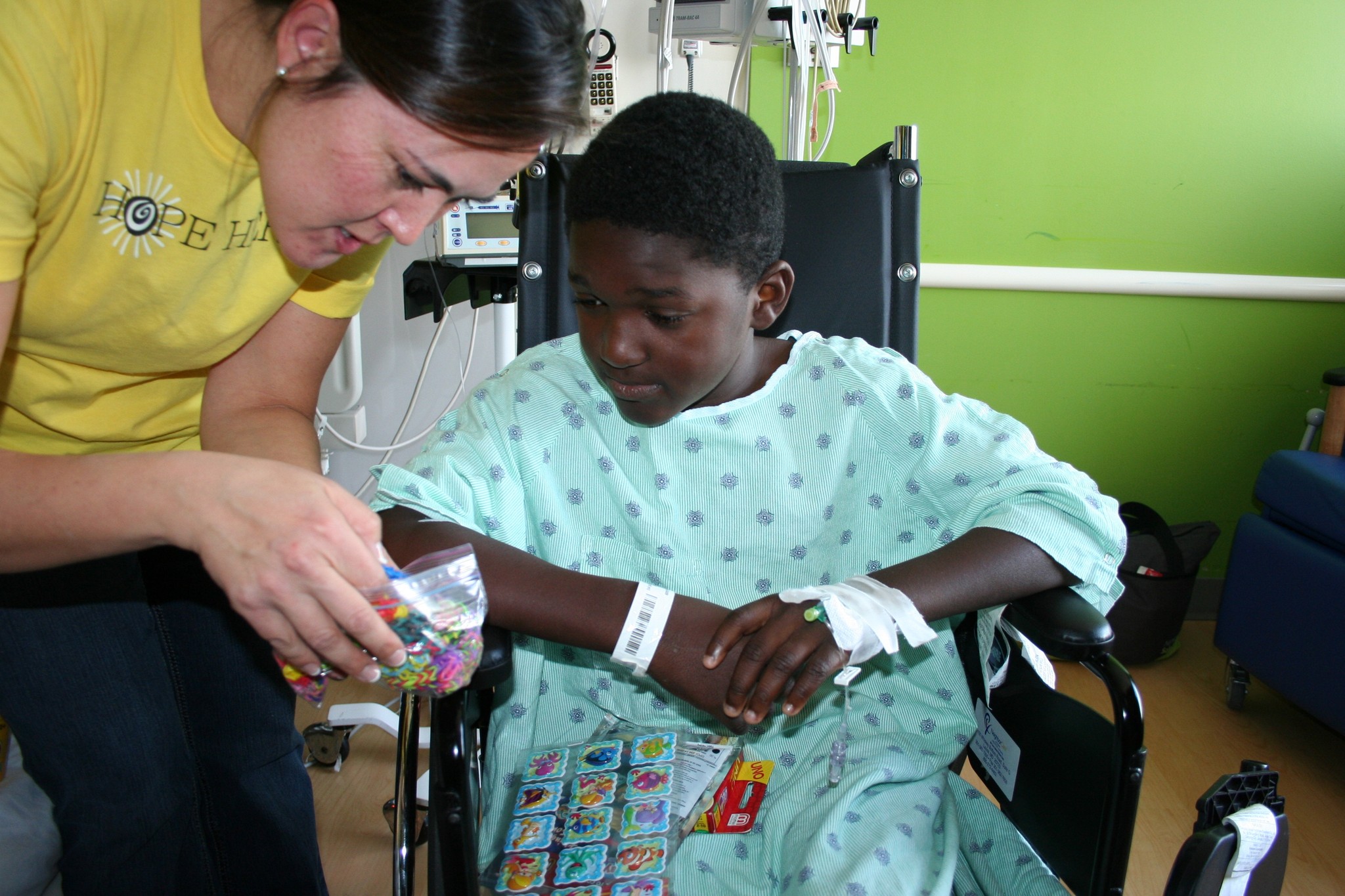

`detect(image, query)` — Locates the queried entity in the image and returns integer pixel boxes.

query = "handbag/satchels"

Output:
[1002,501,1220,666]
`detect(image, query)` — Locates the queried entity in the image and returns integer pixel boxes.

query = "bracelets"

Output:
[608,581,675,675]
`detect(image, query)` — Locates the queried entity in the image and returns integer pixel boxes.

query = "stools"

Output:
[1210,449,1345,735]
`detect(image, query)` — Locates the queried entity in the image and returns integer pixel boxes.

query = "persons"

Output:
[0,0,595,896]
[365,91,1127,896]
